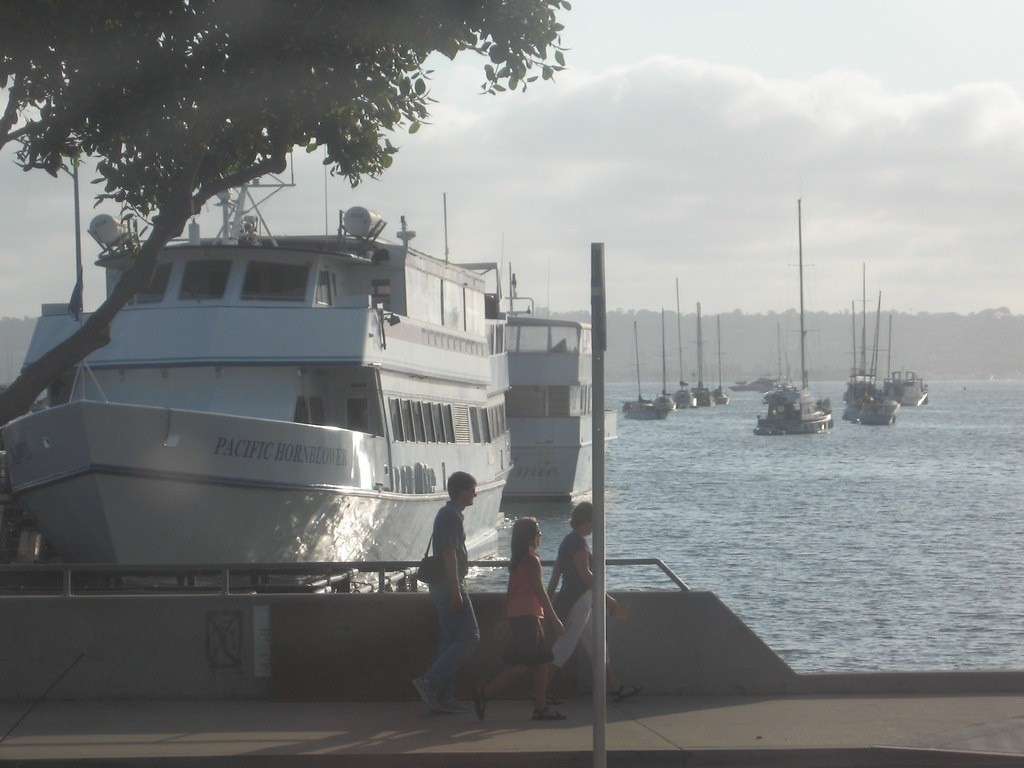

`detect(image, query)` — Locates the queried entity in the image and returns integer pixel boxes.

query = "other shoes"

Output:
[411,678,441,712]
[430,695,472,713]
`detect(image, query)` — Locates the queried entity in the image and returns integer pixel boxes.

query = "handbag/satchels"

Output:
[417,555,433,583]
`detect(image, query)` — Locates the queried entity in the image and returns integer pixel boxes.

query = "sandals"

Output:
[611,684,641,702]
[468,685,486,721]
[545,695,564,705]
[531,706,567,720]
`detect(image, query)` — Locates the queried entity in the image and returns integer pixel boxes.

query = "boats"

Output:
[0,146,516,592]
[504,264,621,499]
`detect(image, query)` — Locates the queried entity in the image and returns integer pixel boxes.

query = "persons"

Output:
[540,502,643,705]
[410,472,481,712]
[473,516,567,720]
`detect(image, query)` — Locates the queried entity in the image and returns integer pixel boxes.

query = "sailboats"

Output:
[751,199,835,436]
[620,259,929,425]
